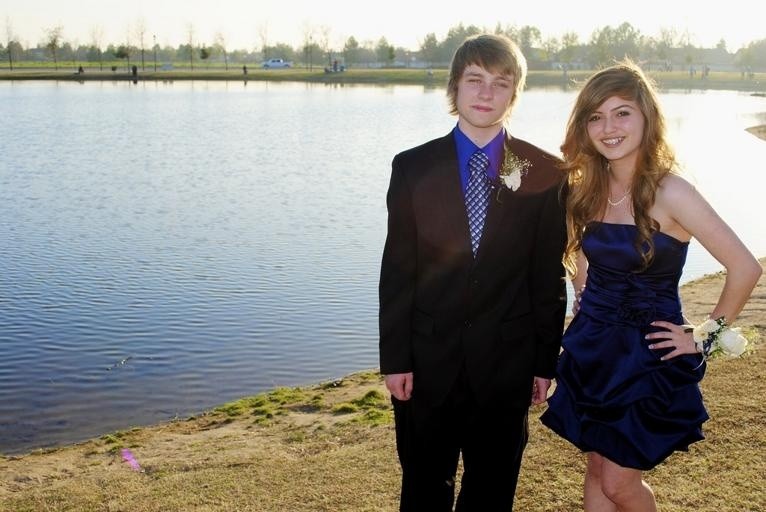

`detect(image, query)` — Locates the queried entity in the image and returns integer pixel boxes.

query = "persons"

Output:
[78,66,85,74]
[378,33,569,512]
[539,64,762,512]
[243,65,248,74]
[324,58,345,73]
[131,64,138,76]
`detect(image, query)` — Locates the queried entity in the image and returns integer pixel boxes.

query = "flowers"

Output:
[499,150,534,192]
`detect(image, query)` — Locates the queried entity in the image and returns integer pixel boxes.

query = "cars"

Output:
[263,59,291,68]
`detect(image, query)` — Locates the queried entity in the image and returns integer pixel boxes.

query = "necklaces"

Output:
[605,162,632,206]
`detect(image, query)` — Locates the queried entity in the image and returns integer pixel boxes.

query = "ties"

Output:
[464,149,492,259]
